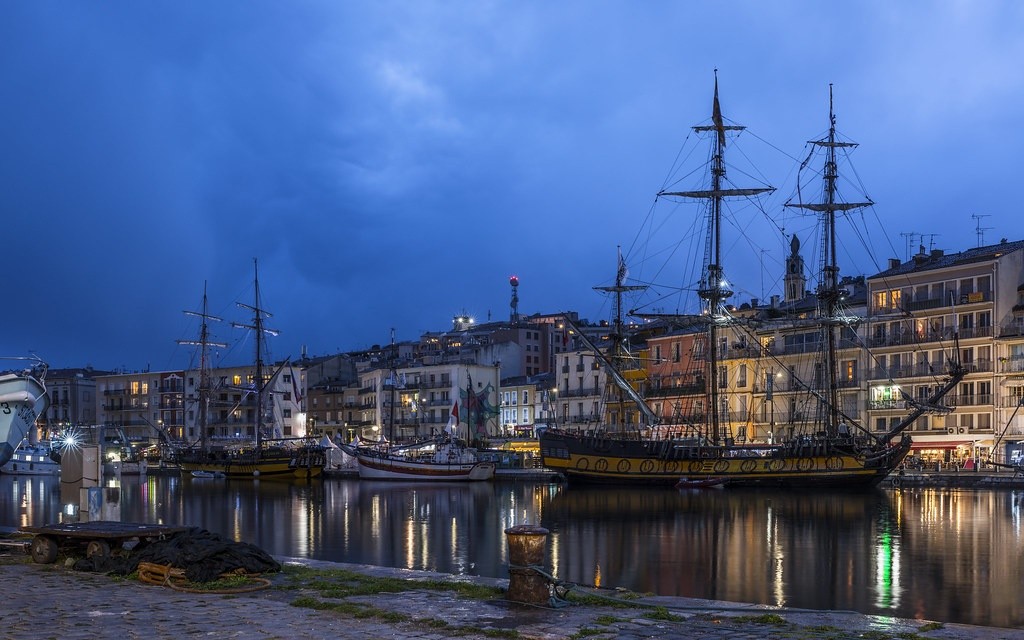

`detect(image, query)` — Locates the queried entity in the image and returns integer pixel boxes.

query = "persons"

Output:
[838,421,848,444]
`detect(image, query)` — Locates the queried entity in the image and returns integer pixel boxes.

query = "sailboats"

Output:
[534,64,976,489]
[1,256,568,482]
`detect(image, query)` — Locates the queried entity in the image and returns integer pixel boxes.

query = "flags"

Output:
[712,77,726,147]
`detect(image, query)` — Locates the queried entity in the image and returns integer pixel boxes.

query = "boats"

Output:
[191,470,227,478]
[0,350,50,469]
